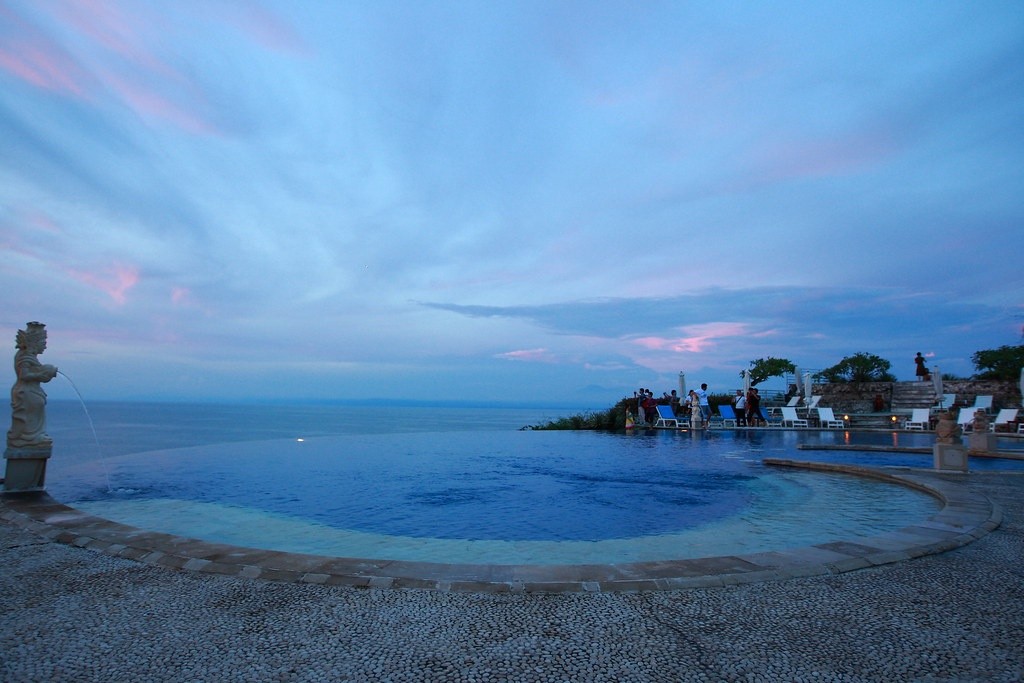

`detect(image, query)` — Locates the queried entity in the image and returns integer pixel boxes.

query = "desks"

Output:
[952,403,970,411]
[1007,421,1024,433]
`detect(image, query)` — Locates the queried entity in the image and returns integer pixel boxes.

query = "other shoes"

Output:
[762,421,767,427]
[702,426,710,432]
[748,422,752,426]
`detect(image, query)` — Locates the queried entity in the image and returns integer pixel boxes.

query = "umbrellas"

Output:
[794,366,804,397]
[678,371,686,416]
[933,365,947,411]
[741,368,751,403]
[803,371,812,418]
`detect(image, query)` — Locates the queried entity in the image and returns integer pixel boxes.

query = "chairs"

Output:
[760,407,782,427]
[957,408,977,432]
[655,405,690,427]
[718,405,748,427]
[766,396,800,413]
[818,408,844,428]
[905,409,930,430]
[968,395,994,414]
[931,394,956,412]
[781,407,808,428]
[990,409,1019,433]
[797,396,822,414]
[703,404,724,427]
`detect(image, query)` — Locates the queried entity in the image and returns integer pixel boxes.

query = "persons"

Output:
[634,388,658,431]
[7,320,53,448]
[731,387,769,428]
[669,383,712,430]
[915,352,927,383]
